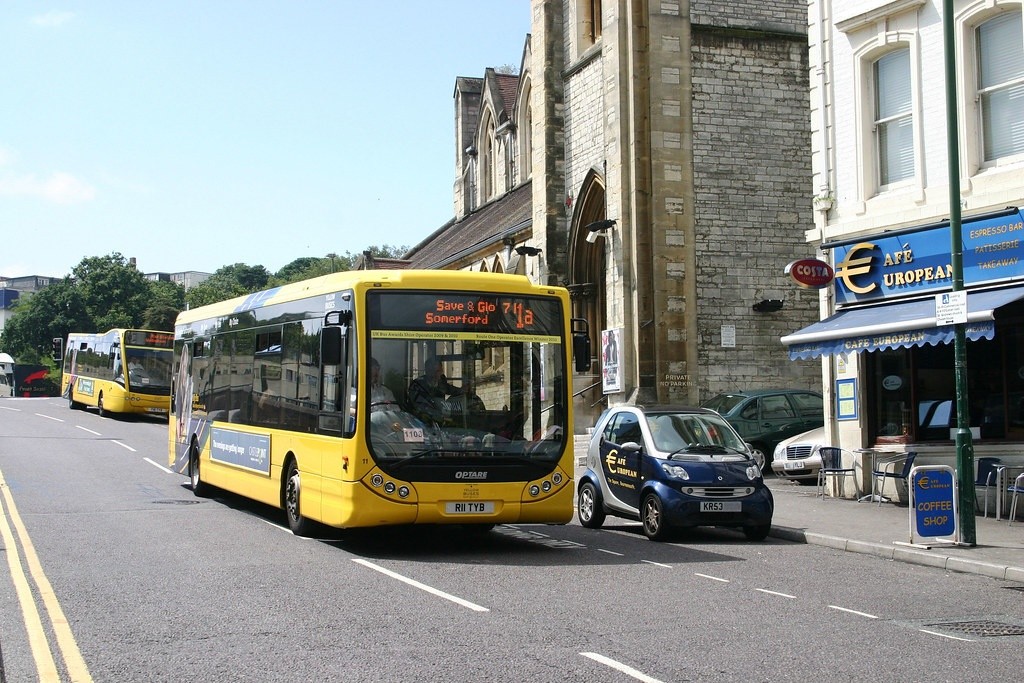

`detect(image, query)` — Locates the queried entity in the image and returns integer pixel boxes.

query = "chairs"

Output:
[1007,473,1024,522]
[817,446,859,501]
[871,450,918,507]
[952,457,1002,518]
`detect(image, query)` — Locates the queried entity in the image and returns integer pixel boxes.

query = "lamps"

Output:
[515,246,541,256]
[585,218,616,234]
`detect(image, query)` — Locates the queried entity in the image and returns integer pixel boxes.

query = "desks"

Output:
[853,448,904,503]
[992,462,1024,522]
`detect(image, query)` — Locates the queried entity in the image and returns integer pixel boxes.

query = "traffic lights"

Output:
[53,339,63,361]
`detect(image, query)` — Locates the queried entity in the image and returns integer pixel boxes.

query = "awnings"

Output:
[780,288,1023,362]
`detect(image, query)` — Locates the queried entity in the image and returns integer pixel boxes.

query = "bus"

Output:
[61,328,174,416]
[169,269,592,537]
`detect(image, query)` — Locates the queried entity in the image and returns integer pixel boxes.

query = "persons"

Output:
[349,358,402,417]
[408,358,467,427]
[127,354,145,372]
[605,331,617,365]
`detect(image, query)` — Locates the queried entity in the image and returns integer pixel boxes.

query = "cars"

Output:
[694,389,823,476]
[576,403,774,541]
[771,424,827,485]
